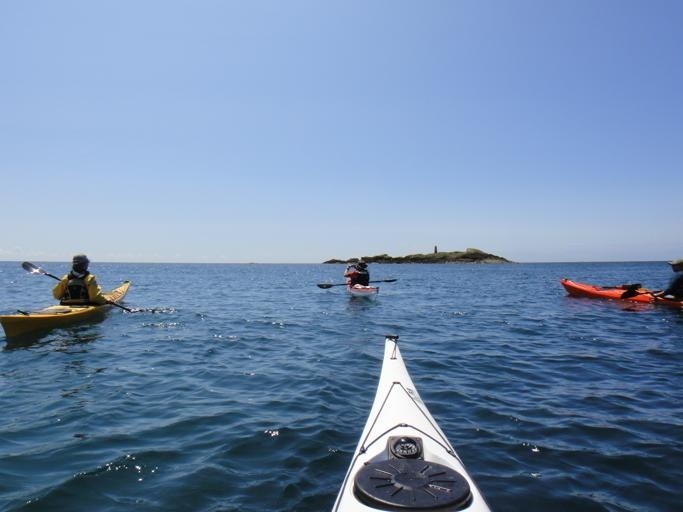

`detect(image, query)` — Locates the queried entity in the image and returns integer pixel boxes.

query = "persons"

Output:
[343,261,370,286]
[51,253,112,307]
[647,259,683,303]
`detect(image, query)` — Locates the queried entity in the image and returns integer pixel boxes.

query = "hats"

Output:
[73,255,90,266]
[667,258,683,265]
[354,260,368,270]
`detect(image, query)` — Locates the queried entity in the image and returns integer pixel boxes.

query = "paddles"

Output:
[22,262,131,311]
[317,277,398,290]
[620,289,663,297]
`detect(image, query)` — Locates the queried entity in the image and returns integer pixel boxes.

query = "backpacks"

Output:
[63,270,89,305]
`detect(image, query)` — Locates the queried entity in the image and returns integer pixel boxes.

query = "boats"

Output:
[559,275,682,307]
[331,333,489,511]
[340,277,378,299]
[0,281,135,339]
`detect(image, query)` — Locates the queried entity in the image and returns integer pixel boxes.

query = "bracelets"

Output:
[653,294,657,296]
